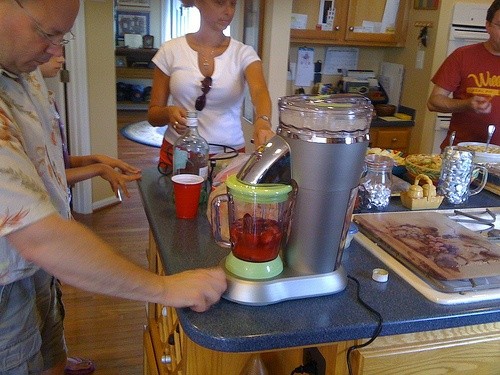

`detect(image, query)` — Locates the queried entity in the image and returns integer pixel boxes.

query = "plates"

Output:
[390,174,412,197]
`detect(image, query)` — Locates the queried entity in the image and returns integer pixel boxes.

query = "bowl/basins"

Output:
[458,141,500,175]
[376,103,397,117]
[391,164,408,178]
[404,153,444,185]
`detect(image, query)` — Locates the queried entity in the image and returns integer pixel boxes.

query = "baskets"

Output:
[406,154,442,187]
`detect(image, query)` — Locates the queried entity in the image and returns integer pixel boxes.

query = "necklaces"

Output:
[202,37,225,69]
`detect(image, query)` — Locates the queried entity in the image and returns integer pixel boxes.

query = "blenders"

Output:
[207,93,377,307]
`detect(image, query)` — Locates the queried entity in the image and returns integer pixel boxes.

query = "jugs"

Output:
[210,174,298,281]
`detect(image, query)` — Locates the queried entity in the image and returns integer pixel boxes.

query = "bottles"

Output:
[422,184,437,197]
[408,186,423,199]
[172,110,209,205]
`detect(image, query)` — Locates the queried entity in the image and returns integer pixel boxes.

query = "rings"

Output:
[175,122,178,125]
[173,125,176,128]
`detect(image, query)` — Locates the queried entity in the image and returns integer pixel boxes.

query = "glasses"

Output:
[15,0,75,48]
[195,76,212,111]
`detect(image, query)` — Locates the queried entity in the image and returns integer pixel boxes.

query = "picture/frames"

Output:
[116,11,150,41]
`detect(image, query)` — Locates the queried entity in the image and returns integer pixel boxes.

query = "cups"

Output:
[437,145,488,205]
[171,173,204,219]
[358,155,394,209]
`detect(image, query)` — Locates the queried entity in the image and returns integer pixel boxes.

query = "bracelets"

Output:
[259,115,268,120]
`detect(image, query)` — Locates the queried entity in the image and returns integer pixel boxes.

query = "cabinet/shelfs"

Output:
[369,118,415,161]
[115,47,158,109]
[290,0,412,48]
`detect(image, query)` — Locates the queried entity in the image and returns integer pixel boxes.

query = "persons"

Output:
[39,53,142,375]
[427,0,500,151]
[147,0,275,177]
[1,0,230,375]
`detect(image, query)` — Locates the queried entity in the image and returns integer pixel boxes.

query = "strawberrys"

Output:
[231,217,281,247]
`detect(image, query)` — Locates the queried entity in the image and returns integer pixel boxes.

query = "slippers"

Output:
[65,357,96,374]
